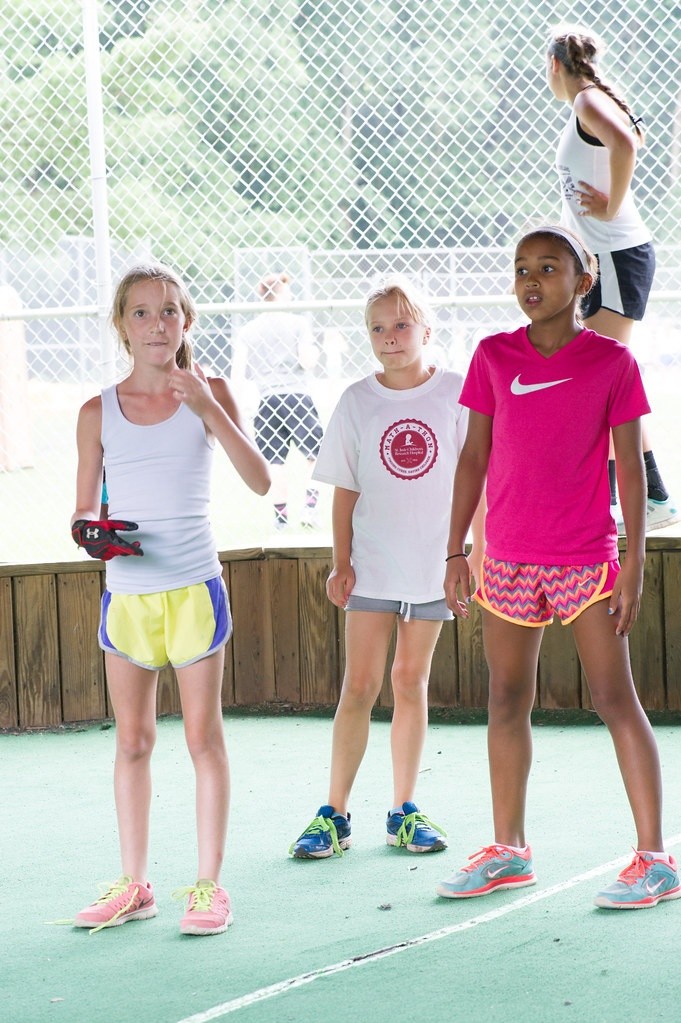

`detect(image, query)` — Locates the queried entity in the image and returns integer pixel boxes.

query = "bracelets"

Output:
[445,554,468,561]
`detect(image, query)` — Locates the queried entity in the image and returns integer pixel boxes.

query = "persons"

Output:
[545,28,680,537]
[433,225,681,910]
[68,260,272,937]
[289,282,484,862]
[240,277,324,530]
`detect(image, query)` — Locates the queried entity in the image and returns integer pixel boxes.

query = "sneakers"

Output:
[385,802,447,855]
[288,806,353,861]
[74,872,159,936]
[172,877,232,937]
[594,847,681,910]
[436,843,537,899]
[615,493,681,538]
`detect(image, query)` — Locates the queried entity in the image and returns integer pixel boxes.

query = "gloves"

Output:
[72,520,146,563]
